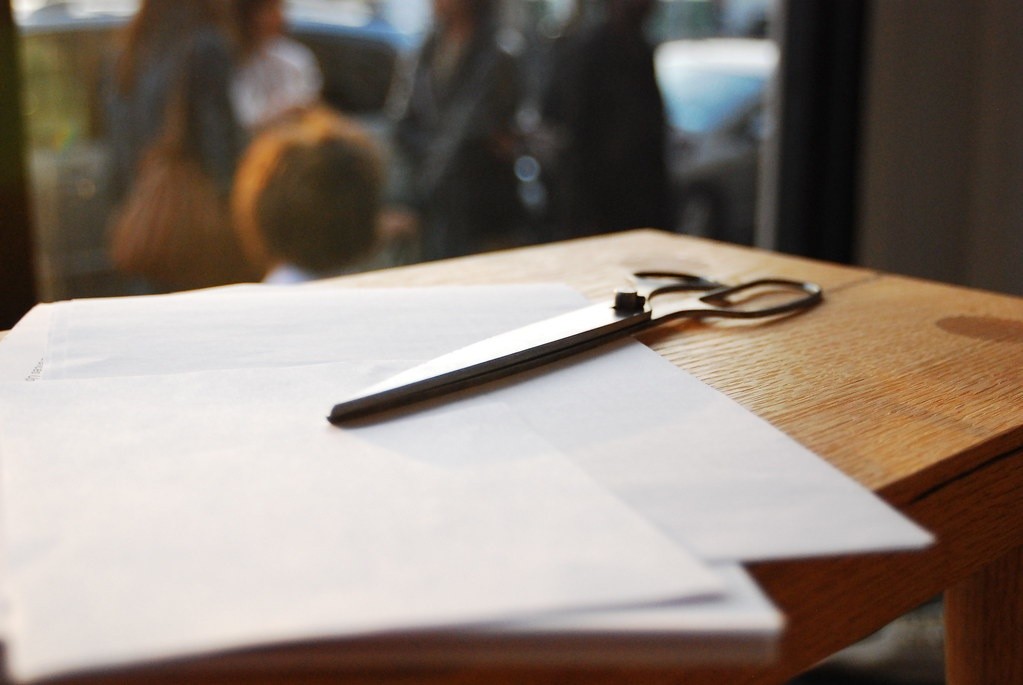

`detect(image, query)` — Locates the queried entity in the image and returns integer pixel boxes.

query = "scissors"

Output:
[327,263,825,424]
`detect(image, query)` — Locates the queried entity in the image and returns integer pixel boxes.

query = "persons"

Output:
[57,0,677,294]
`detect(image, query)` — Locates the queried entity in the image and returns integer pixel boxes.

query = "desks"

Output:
[0,227,1023,685]
